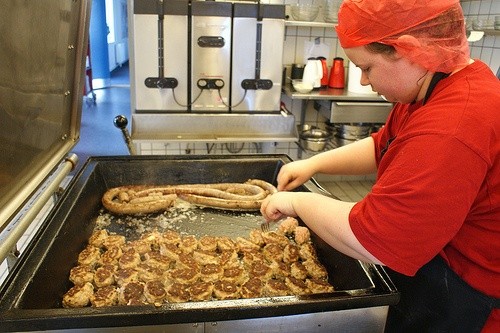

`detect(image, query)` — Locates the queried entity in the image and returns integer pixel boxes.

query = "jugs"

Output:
[302,57,323,88]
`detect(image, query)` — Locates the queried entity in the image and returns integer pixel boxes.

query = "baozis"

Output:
[59,214,333,307]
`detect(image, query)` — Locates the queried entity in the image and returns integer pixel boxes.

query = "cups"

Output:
[329,57,344,88]
[317,57,329,88]
[291,64,304,87]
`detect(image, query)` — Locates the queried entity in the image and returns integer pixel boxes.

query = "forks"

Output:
[261,222,270,233]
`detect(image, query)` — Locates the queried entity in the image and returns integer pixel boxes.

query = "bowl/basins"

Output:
[297,120,384,155]
[290,0,344,23]
[292,79,314,94]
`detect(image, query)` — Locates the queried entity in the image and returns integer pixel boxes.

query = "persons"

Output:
[260,1,500,333]
